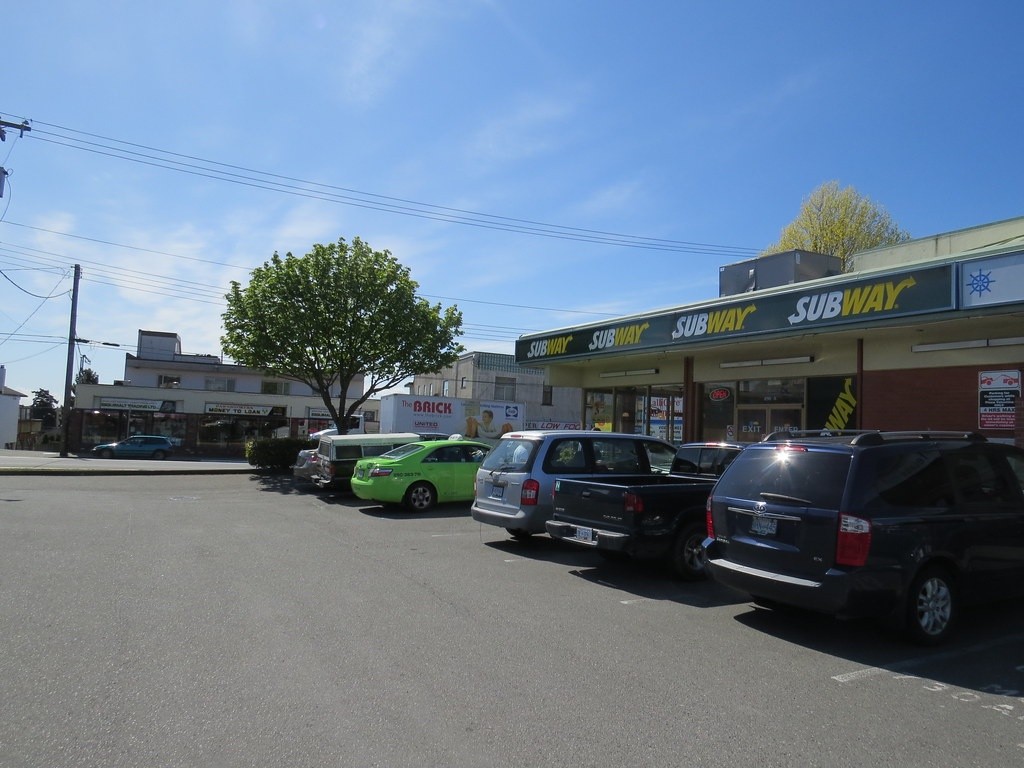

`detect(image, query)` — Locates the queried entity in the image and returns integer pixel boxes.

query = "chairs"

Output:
[594,447,608,473]
[613,448,641,474]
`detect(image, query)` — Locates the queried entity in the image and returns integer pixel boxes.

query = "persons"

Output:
[475,410,503,439]
[578,427,601,460]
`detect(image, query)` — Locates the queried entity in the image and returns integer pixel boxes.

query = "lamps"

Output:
[600,369,659,378]
[910,337,1024,352]
[719,356,814,369]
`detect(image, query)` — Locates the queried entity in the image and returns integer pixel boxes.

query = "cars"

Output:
[90,436,175,460]
[351,439,493,513]
[470,431,681,542]
[293,449,317,480]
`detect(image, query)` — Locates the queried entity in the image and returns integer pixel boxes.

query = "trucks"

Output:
[311,393,526,445]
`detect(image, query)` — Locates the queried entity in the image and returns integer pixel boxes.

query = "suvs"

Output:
[701,428,1024,646]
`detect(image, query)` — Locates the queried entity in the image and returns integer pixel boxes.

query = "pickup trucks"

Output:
[311,432,453,498]
[545,441,756,580]
